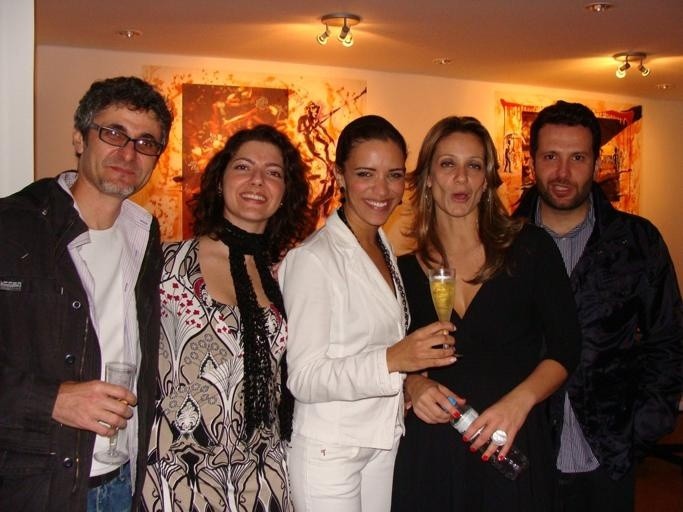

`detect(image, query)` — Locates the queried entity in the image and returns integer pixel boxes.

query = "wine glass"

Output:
[427,268,457,349]
[94,360,136,466]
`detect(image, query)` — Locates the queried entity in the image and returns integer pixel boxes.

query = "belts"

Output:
[88,461,130,488]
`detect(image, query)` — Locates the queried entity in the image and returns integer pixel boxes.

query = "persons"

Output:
[0,76,174,512]
[388,117,581,512]
[505,98,683,512]
[275,113,459,512]
[136,123,307,512]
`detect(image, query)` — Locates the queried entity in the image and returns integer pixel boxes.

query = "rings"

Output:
[491,430,507,447]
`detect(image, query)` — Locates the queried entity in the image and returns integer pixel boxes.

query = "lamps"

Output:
[316,13,362,47]
[612,52,650,79]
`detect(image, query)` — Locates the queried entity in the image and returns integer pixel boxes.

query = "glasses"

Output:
[92,119,166,156]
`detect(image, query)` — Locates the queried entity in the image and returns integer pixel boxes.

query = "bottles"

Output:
[440,396,528,480]
